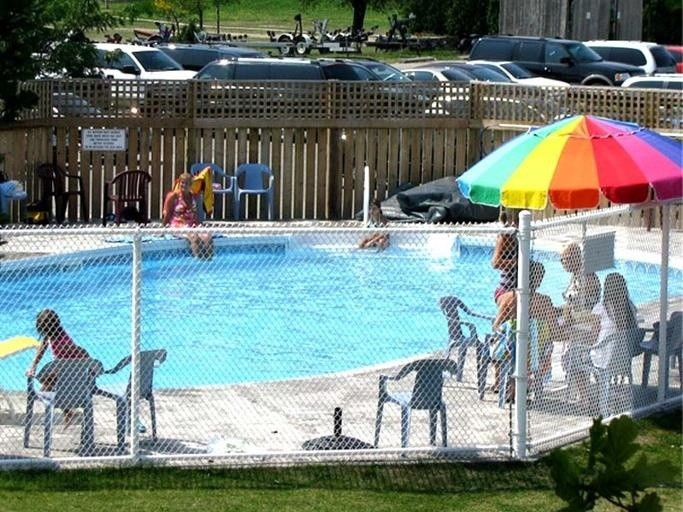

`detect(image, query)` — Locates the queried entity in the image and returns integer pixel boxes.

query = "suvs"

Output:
[30,42,270,165]
[581,40,683,124]
[467,34,645,86]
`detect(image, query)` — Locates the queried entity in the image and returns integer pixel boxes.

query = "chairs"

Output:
[21,348,165,456]
[102,170,153,227]
[374,358,458,457]
[187,162,276,221]
[33,163,88,223]
[439,296,682,416]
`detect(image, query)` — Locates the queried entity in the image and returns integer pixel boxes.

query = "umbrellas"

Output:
[454,113,682,314]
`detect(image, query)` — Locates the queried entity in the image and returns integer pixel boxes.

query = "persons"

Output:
[159,173,212,261]
[489,261,558,406]
[23,309,93,426]
[550,244,601,410]
[561,272,637,411]
[491,206,529,308]
[353,200,390,252]
